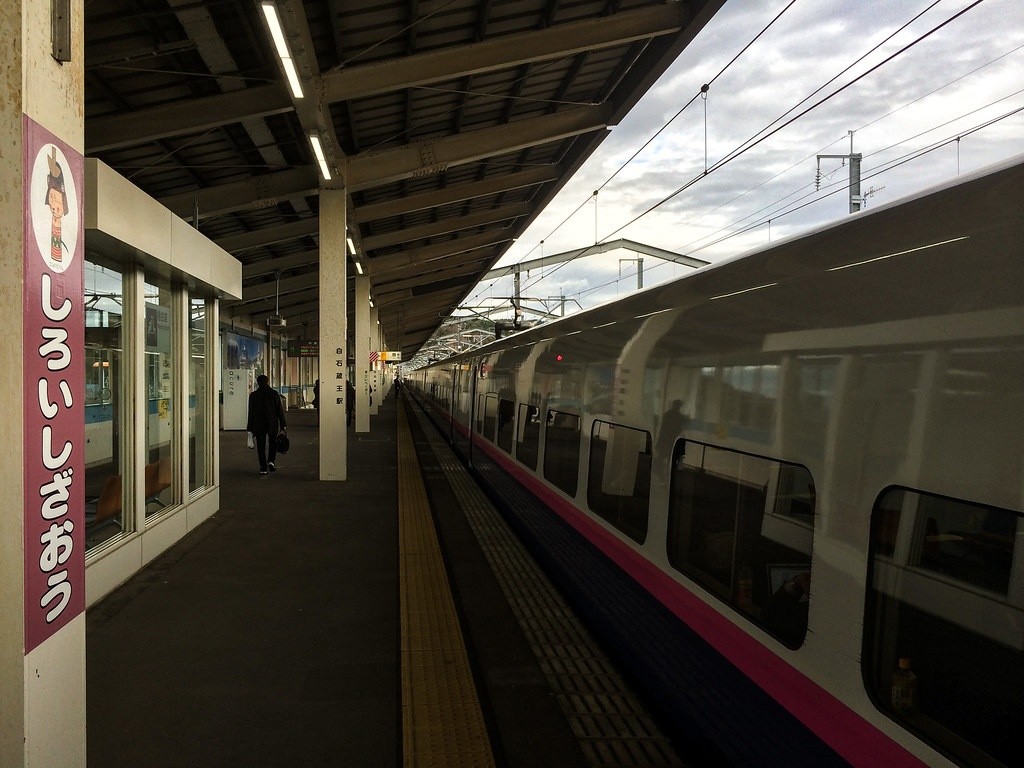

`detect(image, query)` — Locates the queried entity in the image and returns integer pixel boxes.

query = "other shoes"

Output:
[267,461,277,474]
[259,467,268,474]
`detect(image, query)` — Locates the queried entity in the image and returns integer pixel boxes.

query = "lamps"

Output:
[309,130,331,180]
[255,3,305,99]
[339,225,382,325]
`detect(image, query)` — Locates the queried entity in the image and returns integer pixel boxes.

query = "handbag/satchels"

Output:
[276,427,290,454]
[312,399,318,408]
[247,431,255,448]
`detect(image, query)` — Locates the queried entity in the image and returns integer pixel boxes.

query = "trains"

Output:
[398,151,1024,768]
[82,342,196,471]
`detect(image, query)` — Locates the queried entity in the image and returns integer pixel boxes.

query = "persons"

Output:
[395,375,400,399]
[247,374,287,474]
[346,380,356,427]
[312,379,319,426]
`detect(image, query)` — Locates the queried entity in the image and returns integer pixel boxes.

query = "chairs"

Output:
[85,455,184,548]
